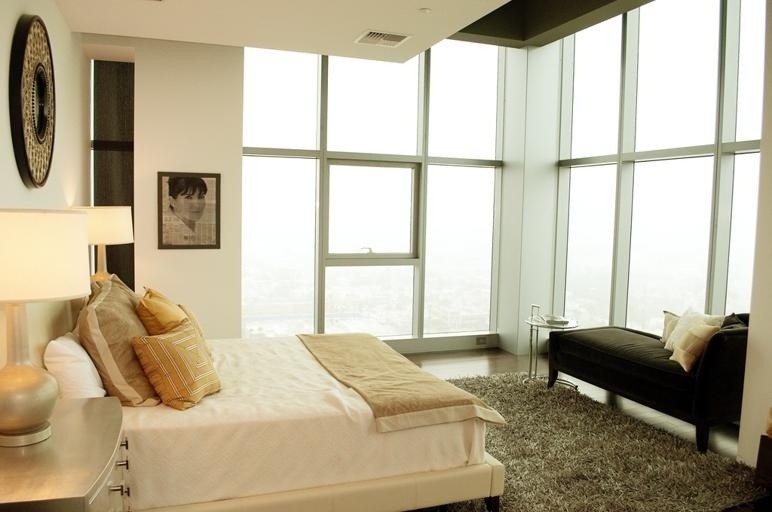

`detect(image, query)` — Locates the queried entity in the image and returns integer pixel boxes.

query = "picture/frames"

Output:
[155,168,225,254]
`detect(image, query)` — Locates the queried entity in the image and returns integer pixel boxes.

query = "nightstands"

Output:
[0,392,138,512]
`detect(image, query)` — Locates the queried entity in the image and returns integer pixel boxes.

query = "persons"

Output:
[163,177,215,245]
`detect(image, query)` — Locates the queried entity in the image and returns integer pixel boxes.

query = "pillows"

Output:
[657,311,746,373]
[133,284,204,366]
[42,328,107,400]
[76,273,164,404]
[130,318,224,414]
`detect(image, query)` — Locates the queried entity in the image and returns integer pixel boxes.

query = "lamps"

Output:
[75,205,136,275]
[0,208,93,447]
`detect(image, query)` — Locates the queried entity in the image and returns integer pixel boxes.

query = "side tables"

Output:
[524,313,580,394]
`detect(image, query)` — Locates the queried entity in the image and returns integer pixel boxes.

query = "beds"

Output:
[507,386,743,512]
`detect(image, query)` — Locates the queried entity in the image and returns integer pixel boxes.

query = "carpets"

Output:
[408,373,772,512]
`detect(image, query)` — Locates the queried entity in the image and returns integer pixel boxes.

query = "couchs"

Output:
[546,309,751,453]
